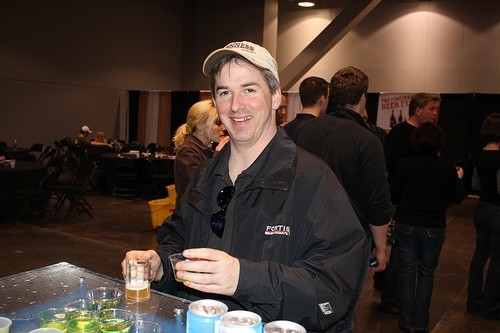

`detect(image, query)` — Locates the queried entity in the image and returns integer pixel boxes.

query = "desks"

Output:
[102,152,177,198]
[0,262,190,333]
[0,161,47,220]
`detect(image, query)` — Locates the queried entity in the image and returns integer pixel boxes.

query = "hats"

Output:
[201,41,279,84]
[81,125,92,134]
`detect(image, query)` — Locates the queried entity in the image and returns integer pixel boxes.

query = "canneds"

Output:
[263,320,307,333]
[219,310,263,333]
[186,299,228,333]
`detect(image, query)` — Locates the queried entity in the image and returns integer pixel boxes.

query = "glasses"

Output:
[210,186,235,238]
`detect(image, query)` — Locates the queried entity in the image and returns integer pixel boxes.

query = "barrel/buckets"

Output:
[166,184,176,210]
[147,197,170,228]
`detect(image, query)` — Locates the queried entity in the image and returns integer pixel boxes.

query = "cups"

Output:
[169,253,199,282]
[28,328,61,333]
[0,317,13,333]
[37,286,163,333]
[124,258,151,303]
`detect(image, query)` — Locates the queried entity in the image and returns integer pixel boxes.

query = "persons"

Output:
[398,110,403,123]
[390,109,396,128]
[276,95,288,127]
[173,99,230,203]
[465,113,500,320]
[121,40,370,333]
[282,66,473,333]
[74,126,113,148]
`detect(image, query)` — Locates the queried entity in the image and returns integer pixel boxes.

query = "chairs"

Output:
[0,137,97,223]
